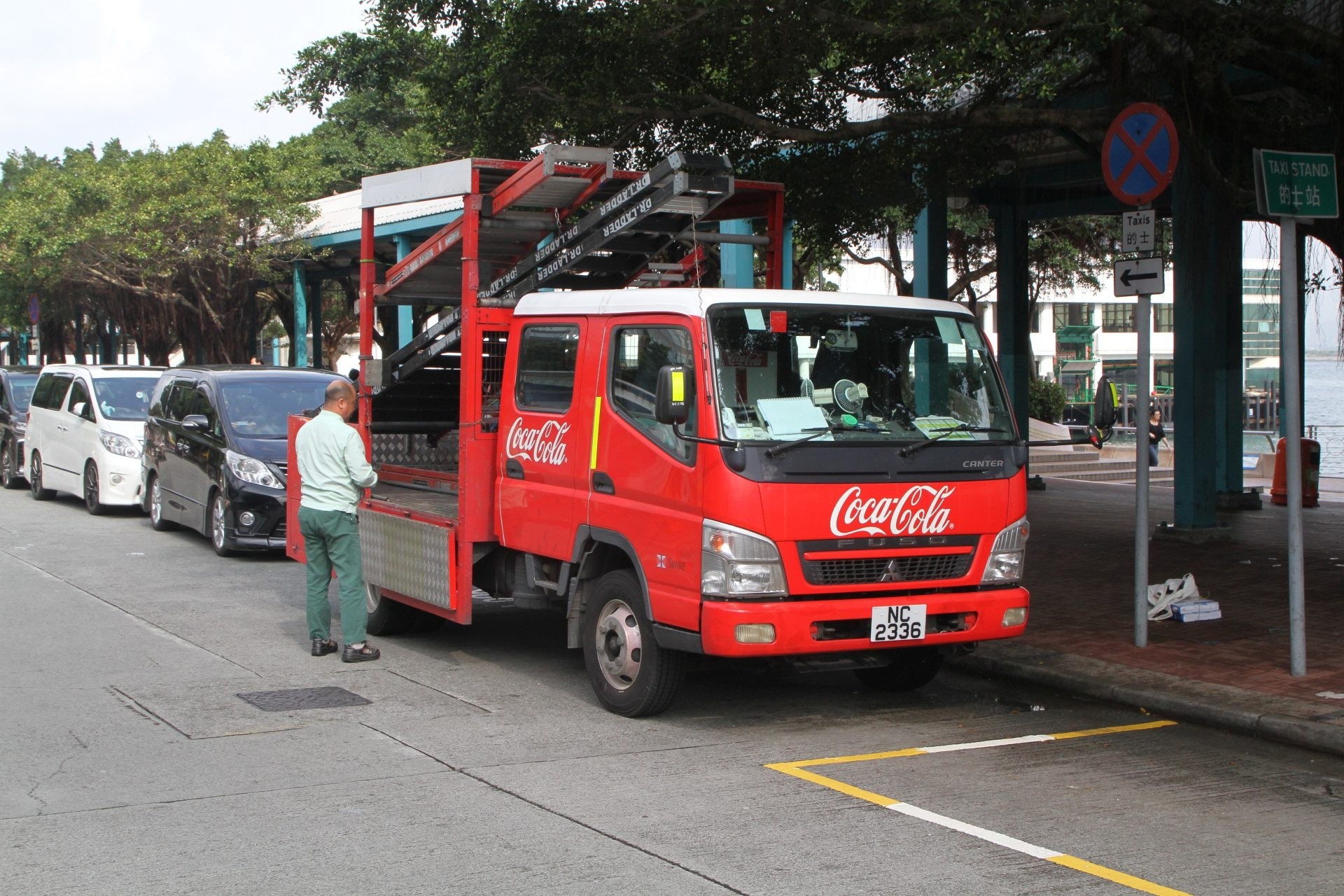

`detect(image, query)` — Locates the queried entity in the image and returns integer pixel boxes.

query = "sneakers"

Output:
[312,637,338,656]
[341,640,380,662]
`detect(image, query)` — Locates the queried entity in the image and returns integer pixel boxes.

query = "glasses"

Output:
[1155,414,1161,416]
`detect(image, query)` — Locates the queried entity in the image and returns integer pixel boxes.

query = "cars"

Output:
[0,365,43,489]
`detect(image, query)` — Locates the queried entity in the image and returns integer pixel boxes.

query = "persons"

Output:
[1147,409,1171,466]
[295,380,381,664]
[250,356,265,365]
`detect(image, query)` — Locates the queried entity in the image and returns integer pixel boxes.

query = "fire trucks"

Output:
[284,142,1120,720]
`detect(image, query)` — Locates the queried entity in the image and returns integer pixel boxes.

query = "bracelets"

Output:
[1167,445,1170,448]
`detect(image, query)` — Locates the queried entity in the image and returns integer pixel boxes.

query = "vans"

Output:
[22,363,171,515]
[136,363,351,559]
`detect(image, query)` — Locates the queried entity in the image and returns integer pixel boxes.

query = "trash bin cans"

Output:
[1268,437,1321,507]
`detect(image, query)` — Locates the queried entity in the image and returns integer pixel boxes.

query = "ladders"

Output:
[302,146,734,422]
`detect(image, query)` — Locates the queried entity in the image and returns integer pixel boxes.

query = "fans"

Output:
[833,379,868,420]
[800,379,833,406]
